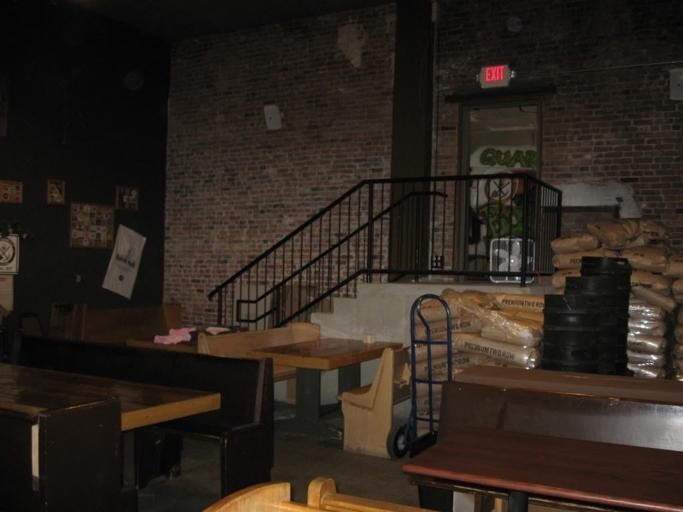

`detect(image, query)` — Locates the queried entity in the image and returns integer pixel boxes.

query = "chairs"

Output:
[35,395,123,508]
[1,300,249,352]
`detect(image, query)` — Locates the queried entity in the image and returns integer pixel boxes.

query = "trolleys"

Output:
[385,293,453,459]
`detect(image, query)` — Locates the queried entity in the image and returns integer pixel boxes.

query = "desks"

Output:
[2,363,222,507]
[246,336,404,437]
[401,425,683,512]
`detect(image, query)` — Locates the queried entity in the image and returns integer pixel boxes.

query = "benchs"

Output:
[211,478,418,511]
[436,363,683,453]
[196,322,323,427]
[9,336,277,497]
[335,346,411,460]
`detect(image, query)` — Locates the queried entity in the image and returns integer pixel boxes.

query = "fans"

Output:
[489,237,536,285]
